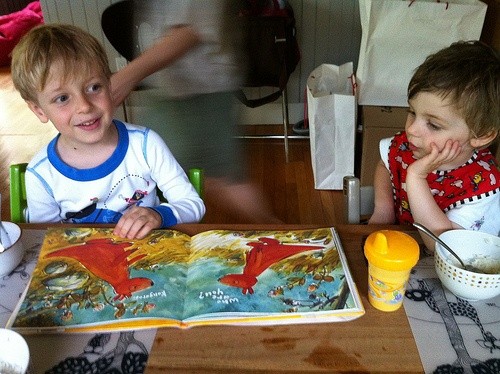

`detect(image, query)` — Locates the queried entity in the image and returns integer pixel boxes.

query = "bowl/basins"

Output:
[0,221,23,276]
[434,229,500,302]
[0,328,30,374]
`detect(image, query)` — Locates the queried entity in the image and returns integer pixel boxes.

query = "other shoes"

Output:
[292,119,309,133]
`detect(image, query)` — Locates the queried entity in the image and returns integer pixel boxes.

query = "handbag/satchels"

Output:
[307,61,356,190]
[357,0,488,107]
[226,0,301,108]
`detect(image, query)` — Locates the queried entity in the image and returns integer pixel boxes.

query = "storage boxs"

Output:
[358,104,410,185]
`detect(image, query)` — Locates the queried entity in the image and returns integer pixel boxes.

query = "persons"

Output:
[110,0,288,228]
[369,40,500,250]
[10,24,207,240]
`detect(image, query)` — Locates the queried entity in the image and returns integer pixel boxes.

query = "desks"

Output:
[0,223,435,374]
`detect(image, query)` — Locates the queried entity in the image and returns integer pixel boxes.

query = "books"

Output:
[8,227,365,335]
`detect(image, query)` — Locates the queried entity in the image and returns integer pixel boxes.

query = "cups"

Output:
[363,230,419,312]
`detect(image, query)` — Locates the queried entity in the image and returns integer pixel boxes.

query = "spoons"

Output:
[0,221,10,248]
[413,222,485,274]
